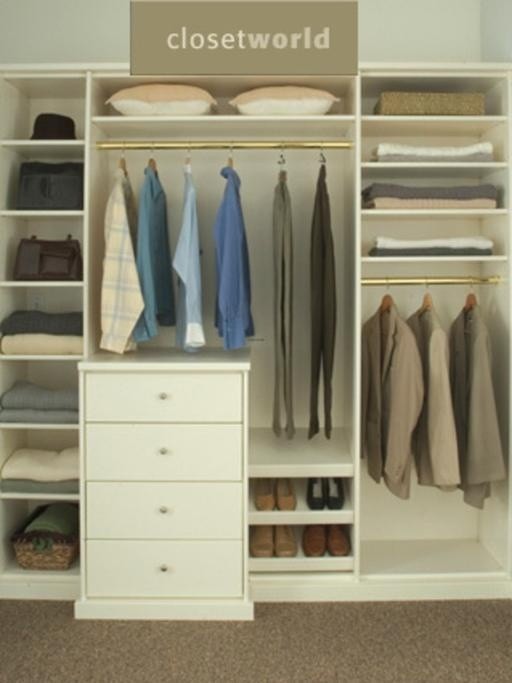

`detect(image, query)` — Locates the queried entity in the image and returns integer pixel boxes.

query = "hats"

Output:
[30,114,76,140]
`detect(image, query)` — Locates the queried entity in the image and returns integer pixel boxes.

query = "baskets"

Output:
[11,505,76,570]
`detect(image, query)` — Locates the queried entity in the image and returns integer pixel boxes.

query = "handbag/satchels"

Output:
[18,163,82,209]
[14,234,83,281]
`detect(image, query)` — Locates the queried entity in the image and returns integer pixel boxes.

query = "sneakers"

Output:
[303,526,325,557]
[327,525,349,556]
[276,479,296,510]
[275,526,297,556]
[307,477,324,509]
[254,477,275,509]
[325,477,344,510]
[251,525,274,556]
